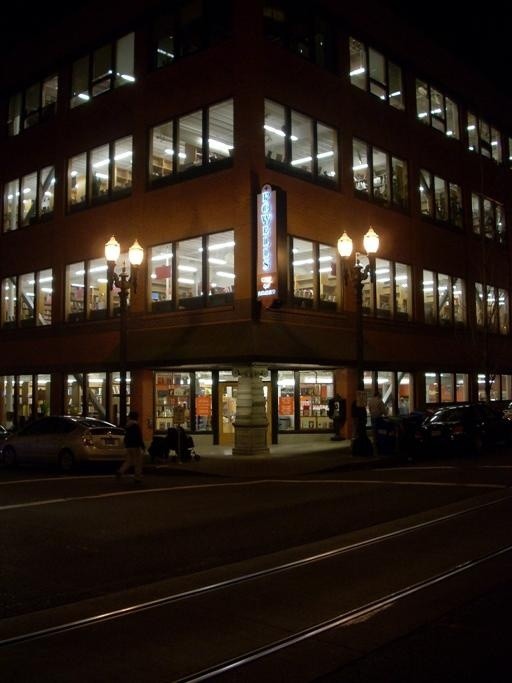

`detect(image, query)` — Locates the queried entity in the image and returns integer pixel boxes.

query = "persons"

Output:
[350,390,367,439]
[39,409,48,418]
[114,410,147,481]
[400,397,409,415]
[367,390,389,448]
[45,407,50,416]
[80,395,89,417]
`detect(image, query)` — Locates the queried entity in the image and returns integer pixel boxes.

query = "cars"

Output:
[374,401,512,459]
[7,416,126,473]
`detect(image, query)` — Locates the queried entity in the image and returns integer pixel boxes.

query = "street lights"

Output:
[338,227,382,457]
[105,233,144,426]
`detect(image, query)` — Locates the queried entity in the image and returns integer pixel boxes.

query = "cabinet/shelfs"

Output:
[354,160,505,242]
[6,279,197,324]
[294,272,463,323]
[72,142,230,204]
[5,373,190,430]
